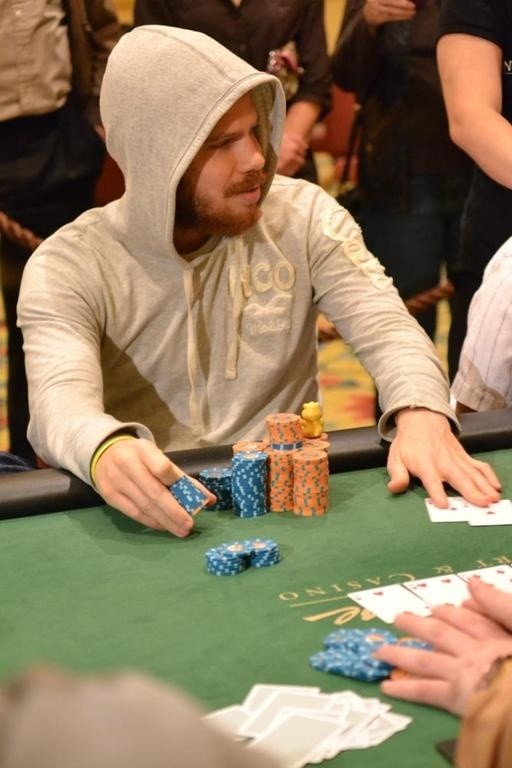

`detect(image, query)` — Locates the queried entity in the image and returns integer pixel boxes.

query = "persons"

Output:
[18,20,504,538]
[370,566,512,768]
[18,20,504,527]
[1,1,120,473]
[133,2,333,186]
[333,0,511,424]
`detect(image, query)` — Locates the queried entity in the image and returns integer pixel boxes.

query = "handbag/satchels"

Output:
[472,654,512,690]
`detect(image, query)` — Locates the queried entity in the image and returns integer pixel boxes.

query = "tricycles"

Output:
[427,496,512,526]
[345,565,511,626]
[197,682,412,768]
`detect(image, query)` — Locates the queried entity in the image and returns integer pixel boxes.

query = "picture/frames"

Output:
[1,406,512,767]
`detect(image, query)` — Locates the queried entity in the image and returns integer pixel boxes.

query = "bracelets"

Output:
[472,654,512,690]
